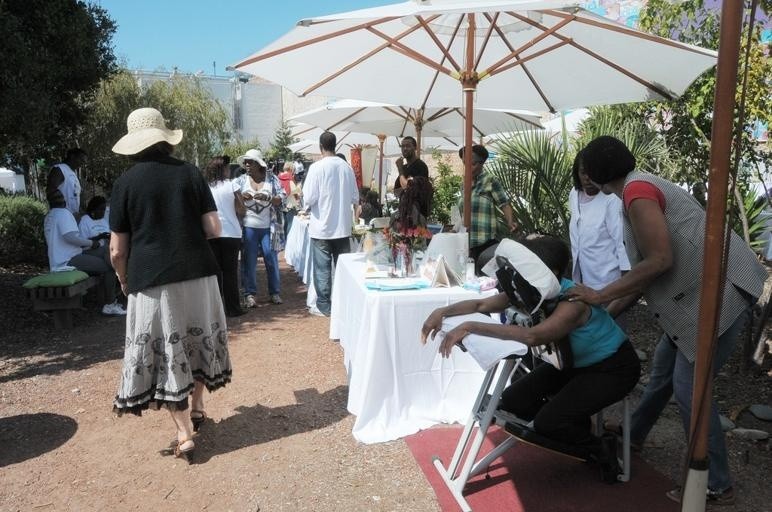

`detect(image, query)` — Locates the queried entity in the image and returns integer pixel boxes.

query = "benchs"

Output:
[28,275,102,330]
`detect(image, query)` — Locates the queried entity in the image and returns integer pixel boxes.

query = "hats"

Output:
[111,108,184,156]
[237,149,267,172]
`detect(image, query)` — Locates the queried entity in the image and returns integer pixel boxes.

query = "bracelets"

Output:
[265,194,273,201]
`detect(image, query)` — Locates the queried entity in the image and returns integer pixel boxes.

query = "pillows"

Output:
[22,270,90,288]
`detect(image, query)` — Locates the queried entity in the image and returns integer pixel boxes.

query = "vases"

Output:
[395,252,410,278]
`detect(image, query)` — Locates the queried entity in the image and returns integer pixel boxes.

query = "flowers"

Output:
[383,221,432,256]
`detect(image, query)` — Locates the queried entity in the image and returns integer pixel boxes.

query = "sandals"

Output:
[595,414,644,451]
[665,485,735,507]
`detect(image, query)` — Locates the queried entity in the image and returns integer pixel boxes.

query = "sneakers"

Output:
[272,294,282,305]
[101,302,127,317]
[244,295,257,310]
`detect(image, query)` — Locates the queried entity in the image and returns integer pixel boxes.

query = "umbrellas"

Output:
[283,141,377,156]
[289,122,388,204]
[224,0,720,234]
[283,98,547,159]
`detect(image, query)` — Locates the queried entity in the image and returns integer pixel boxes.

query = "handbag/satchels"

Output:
[269,178,285,253]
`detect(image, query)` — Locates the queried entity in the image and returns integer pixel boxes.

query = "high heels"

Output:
[188,410,207,432]
[174,438,194,465]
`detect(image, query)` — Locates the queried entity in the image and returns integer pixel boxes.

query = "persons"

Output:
[568,147,632,291]
[78,195,110,249]
[206,155,247,318]
[421,234,641,487]
[45,147,88,215]
[236,149,287,309]
[458,145,519,260]
[109,108,221,466]
[43,189,128,315]
[355,190,383,226]
[301,132,360,317]
[393,136,429,198]
[559,134,768,506]
